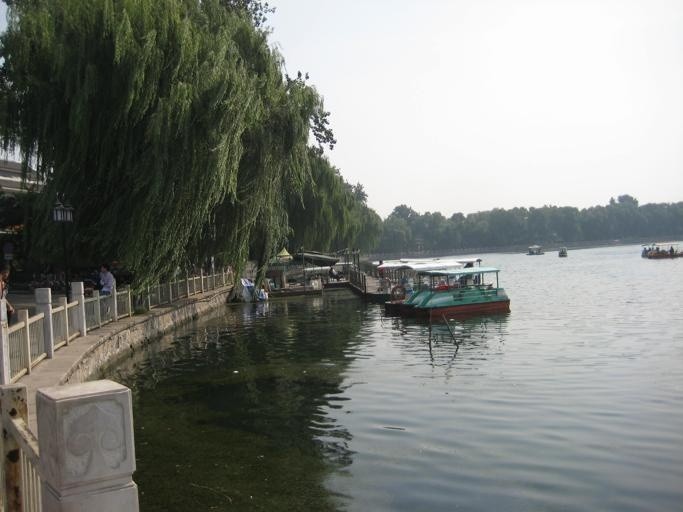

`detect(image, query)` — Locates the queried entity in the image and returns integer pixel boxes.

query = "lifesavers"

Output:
[392,286,406,300]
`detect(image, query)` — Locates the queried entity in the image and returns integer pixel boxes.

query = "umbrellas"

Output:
[270,246,293,272]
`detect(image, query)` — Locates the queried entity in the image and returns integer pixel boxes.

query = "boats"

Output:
[640,242,683,258]
[526,246,544,255]
[301,261,358,282]
[558,247,567,257]
[365,255,511,319]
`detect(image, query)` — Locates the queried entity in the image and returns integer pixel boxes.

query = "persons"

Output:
[329,268,340,281]
[100,263,116,295]
[643,247,674,255]
[0,264,14,324]
[377,260,384,291]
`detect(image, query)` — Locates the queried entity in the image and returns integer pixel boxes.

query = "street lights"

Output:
[52,190,75,299]
[337,247,360,282]
